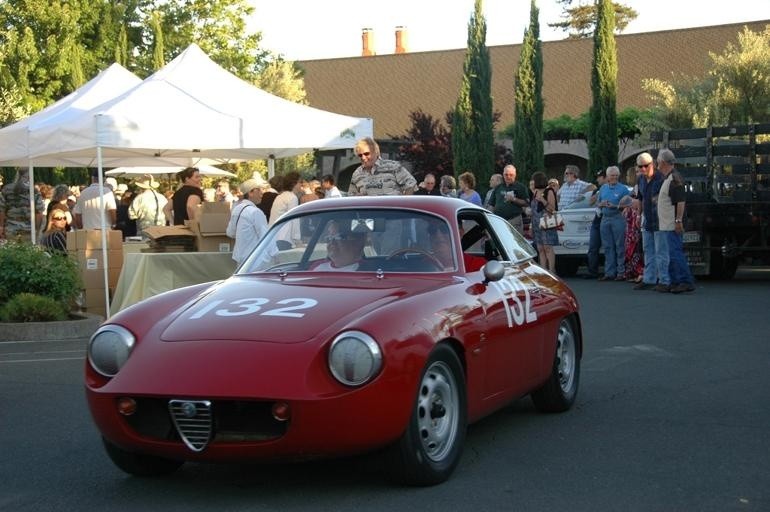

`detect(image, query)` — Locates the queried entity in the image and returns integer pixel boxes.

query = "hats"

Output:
[103,177,117,192]
[240,179,270,195]
[68,195,76,203]
[135,174,160,189]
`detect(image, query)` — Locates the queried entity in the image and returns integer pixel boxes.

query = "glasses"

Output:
[428,225,461,234]
[52,216,67,221]
[325,235,358,242]
[358,150,376,158]
[638,162,652,169]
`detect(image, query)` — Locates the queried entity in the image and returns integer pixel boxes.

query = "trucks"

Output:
[648,121,770,281]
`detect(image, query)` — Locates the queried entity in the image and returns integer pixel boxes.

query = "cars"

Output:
[540,186,636,275]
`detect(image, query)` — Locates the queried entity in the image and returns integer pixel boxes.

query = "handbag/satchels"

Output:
[539,208,565,231]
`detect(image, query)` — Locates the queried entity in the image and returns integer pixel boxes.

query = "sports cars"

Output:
[82,194,583,485]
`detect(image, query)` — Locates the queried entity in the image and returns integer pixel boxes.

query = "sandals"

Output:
[627,275,643,284]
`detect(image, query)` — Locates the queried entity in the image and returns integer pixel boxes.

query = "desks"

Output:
[110,244,379,320]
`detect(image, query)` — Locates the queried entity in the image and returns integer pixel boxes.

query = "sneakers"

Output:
[613,276,625,281]
[598,274,616,281]
[632,281,657,290]
[651,283,680,292]
[671,284,696,294]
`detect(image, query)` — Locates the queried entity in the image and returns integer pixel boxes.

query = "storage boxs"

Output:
[67,229,125,320]
[188,202,234,251]
